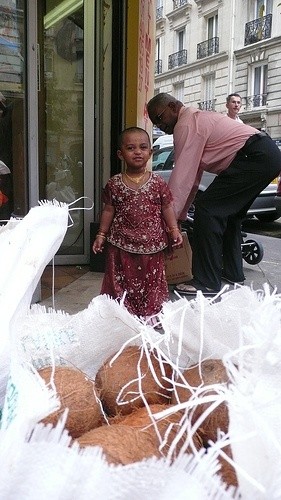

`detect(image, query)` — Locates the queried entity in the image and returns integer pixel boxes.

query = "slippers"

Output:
[221,276,246,285]
[173,278,221,297]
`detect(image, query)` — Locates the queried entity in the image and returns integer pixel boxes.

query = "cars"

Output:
[153,133,281,226]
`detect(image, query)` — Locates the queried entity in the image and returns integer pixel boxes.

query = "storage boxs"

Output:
[164,231,193,285]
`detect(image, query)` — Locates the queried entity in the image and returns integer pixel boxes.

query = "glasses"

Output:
[152,106,169,128]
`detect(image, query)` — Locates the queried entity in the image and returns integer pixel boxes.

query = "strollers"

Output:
[179,200,263,266]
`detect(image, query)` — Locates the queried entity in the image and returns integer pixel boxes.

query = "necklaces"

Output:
[124,169,146,184]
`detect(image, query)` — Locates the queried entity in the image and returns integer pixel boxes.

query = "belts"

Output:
[244,132,268,149]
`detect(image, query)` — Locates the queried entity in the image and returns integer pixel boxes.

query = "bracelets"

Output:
[168,225,179,231]
[95,231,106,239]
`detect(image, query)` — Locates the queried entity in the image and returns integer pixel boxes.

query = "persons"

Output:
[92,127,184,329]
[147,92,281,295]
[224,94,243,124]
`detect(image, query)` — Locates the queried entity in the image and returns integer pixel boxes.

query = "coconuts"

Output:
[33,343,238,493]
